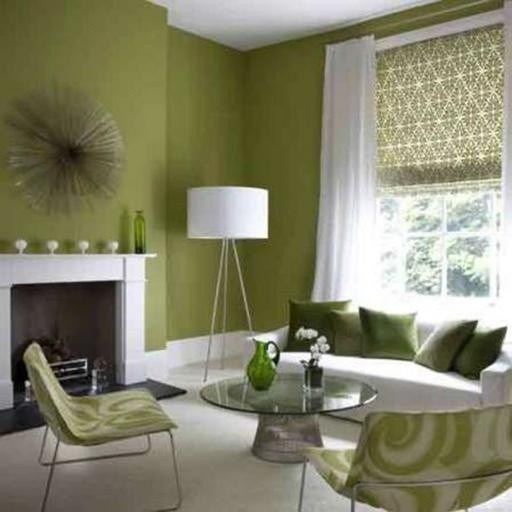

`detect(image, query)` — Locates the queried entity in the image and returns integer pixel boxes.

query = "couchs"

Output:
[244,321,512,423]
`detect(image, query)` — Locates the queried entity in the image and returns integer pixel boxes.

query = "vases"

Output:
[306,367,323,389]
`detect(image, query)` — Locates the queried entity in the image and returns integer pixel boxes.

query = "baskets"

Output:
[13,350,89,387]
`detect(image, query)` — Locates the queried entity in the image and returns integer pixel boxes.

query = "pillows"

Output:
[328,309,364,357]
[452,326,508,379]
[285,298,352,352]
[411,319,478,368]
[359,307,419,360]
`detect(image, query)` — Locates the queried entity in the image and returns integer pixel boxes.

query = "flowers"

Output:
[296,326,330,368]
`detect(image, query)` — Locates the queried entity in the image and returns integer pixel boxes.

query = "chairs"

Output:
[21,342,182,512]
[299,404,512,512]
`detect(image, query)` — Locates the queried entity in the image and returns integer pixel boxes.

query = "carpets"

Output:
[0,377,187,438]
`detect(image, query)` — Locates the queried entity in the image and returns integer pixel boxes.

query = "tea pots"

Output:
[246,338,280,391]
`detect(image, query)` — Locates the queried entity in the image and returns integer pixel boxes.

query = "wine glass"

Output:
[108,241,118,253]
[47,240,58,255]
[15,239,27,255]
[79,240,89,254]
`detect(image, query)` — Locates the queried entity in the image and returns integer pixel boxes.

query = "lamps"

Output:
[187,185,270,382]
